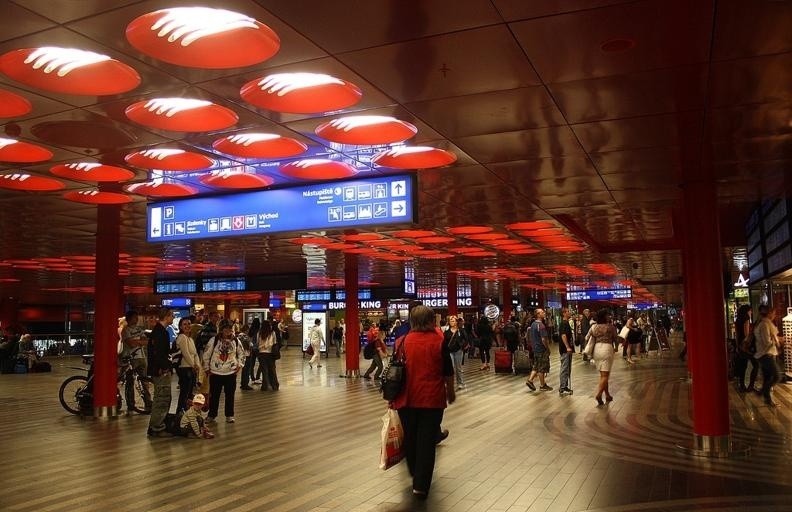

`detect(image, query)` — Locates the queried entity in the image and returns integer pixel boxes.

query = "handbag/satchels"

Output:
[272,343,280,359]
[584,336,596,355]
[619,326,630,340]
[503,322,518,340]
[171,341,182,366]
[382,361,406,400]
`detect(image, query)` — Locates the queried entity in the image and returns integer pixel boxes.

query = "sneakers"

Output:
[559,387,573,395]
[433,429,449,444]
[225,416,235,423]
[204,416,216,423]
[526,380,553,391]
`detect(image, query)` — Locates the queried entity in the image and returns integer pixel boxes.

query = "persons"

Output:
[393,306,456,496]
[17,334,42,373]
[527,307,649,405]
[735,303,785,407]
[307,318,388,379]
[438,315,522,388]
[121,307,288,440]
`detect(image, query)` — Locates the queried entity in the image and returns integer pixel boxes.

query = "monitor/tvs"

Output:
[400,279,417,299]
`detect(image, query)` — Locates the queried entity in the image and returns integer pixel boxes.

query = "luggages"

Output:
[495,345,512,373]
[514,345,532,375]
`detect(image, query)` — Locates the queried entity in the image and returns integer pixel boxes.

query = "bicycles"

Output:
[59,347,155,414]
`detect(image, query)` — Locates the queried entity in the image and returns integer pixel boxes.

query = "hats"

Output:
[193,394,205,405]
[219,319,234,334]
